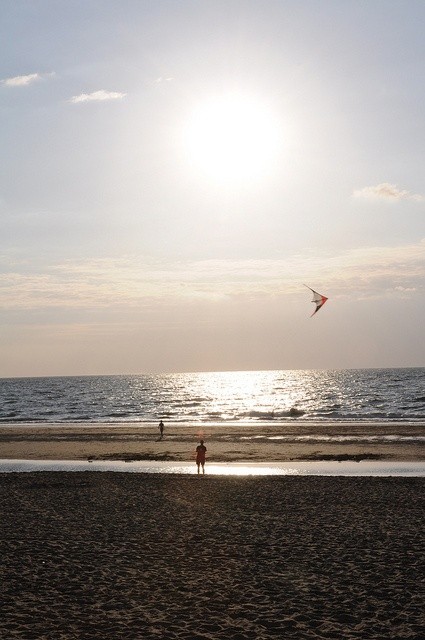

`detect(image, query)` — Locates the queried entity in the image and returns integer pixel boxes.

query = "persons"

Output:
[196,440,207,475]
[158,420,164,439]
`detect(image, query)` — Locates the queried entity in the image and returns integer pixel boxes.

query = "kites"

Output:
[304,284,329,318]
